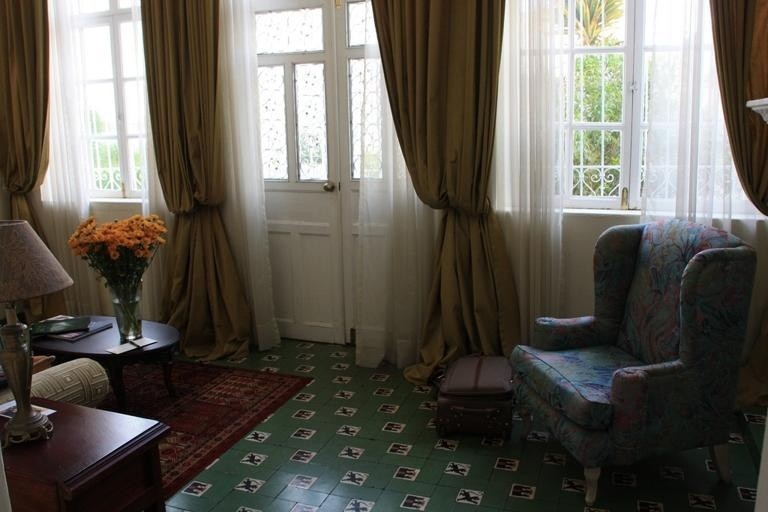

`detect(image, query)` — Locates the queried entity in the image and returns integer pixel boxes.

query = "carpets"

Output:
[95,356,314,500]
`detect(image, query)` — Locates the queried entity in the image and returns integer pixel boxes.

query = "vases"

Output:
[102,277,143,342]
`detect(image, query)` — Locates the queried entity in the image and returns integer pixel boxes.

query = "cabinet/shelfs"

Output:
[0,397,170,512]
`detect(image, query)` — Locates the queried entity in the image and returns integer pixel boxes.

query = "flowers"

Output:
[66,213,169,335]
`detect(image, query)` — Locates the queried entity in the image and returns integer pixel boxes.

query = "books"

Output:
[39,313,112,342]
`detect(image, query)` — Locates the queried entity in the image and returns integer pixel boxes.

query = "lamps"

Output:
[0,220,75,446]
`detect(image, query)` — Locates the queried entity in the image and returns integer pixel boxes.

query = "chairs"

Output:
[508,216,757,507]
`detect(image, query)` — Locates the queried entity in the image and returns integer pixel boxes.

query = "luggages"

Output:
[435,354,515,442]
